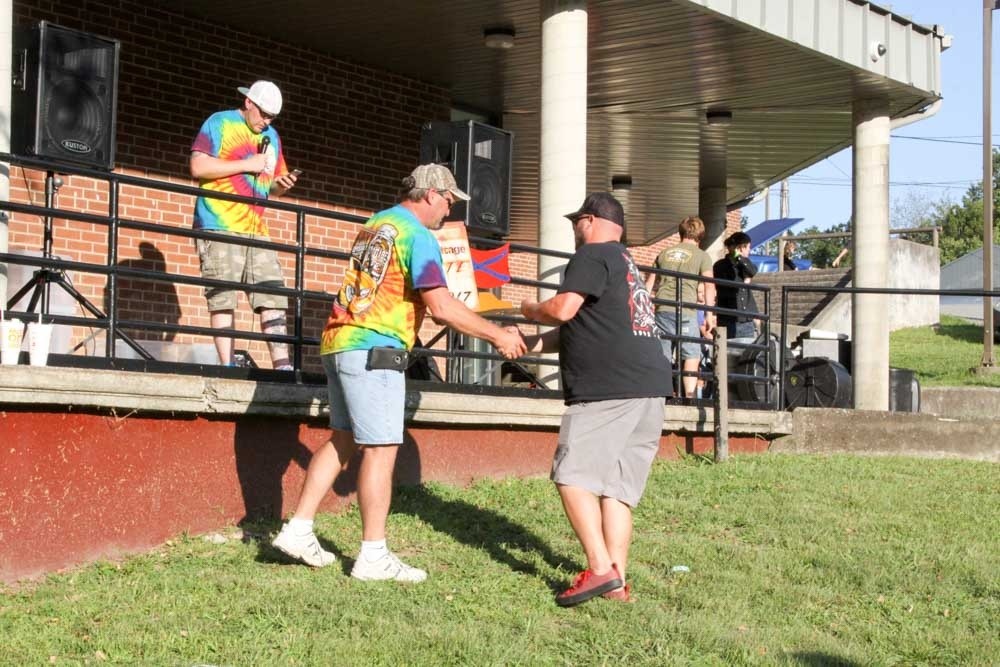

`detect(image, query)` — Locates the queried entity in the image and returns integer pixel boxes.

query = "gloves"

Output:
[731,248,741,259]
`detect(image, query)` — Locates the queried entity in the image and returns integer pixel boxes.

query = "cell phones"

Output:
[289,168,304,177]
[366,346,407,371]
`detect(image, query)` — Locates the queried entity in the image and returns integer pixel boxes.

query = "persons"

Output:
[189,79,298,371]
[785,242,813,272]
[270,160,530,584]
[714,231,762,339]
[831,240,853,266]
[500,192,674,607]
[647,215,717,399]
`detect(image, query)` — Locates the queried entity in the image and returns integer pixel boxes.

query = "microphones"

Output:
[255,136,271,177]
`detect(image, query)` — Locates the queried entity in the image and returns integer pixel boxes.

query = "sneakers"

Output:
[350,545,427,585]
[600,583,630,604]
[555,563,622,607]
[271,522,335,568]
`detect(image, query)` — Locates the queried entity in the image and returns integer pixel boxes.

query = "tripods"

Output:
[7,173,161,362]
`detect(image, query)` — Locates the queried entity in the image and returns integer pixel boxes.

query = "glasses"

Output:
[427,188,455,209]
[572,215,598,224]
[251,101,278,120]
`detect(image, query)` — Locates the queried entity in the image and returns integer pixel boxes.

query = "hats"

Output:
[725,232,751,245]
[237,80,282,117]
[411,163,471,201]
[563,192,624,226]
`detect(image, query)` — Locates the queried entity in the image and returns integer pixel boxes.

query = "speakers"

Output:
[418,118,515,237]
[12,20,122,172]
